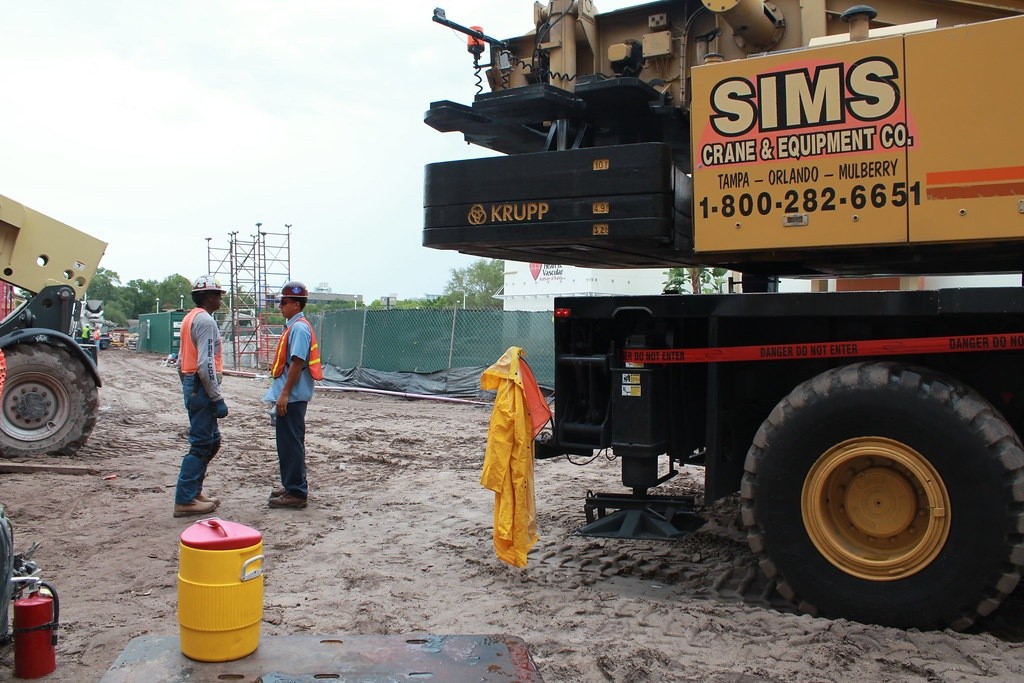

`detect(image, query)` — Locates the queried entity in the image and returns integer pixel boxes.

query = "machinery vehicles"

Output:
[0,193,109,459]
[421,0,1024,633]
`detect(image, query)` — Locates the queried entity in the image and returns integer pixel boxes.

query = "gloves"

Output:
[215,399,229,419]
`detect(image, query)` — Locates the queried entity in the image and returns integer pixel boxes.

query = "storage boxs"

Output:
[79,344,99,365]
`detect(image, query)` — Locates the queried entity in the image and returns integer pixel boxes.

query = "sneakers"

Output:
[268,487,308,509]
[173,493,221,517]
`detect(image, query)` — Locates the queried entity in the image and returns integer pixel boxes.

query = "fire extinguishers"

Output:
[10,576,60,678]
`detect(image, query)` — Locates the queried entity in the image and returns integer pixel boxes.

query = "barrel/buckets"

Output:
[178,517,263,662]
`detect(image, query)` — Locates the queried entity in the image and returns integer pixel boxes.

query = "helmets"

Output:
[192,274,227,295]
[276,281,309,298]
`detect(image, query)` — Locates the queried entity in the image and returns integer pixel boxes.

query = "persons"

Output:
[94,325,101,353]
[174,275,229,518]
[82,325,90,343]
[261,282,325,510]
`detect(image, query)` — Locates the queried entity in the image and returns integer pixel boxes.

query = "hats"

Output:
[86,324,90,327]
